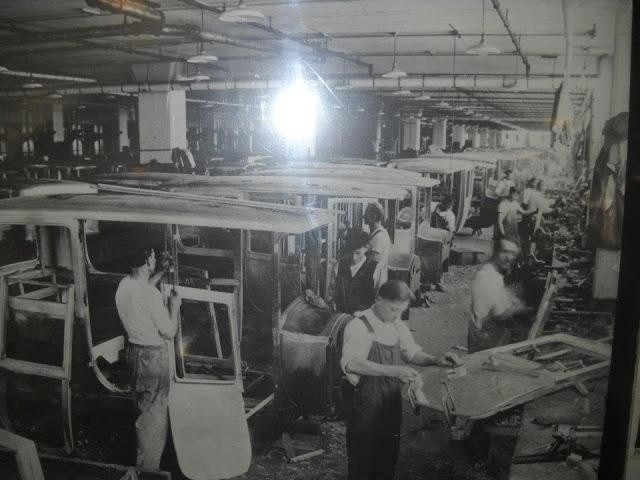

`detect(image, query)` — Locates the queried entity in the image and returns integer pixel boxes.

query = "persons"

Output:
[113,243,183,473]
[339,279,461,479]
[348,203,390,306]
[429,169,548,251]
[463,237,525,354]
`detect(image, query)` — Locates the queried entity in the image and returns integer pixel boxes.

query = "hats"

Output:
[341,226,369,250]
